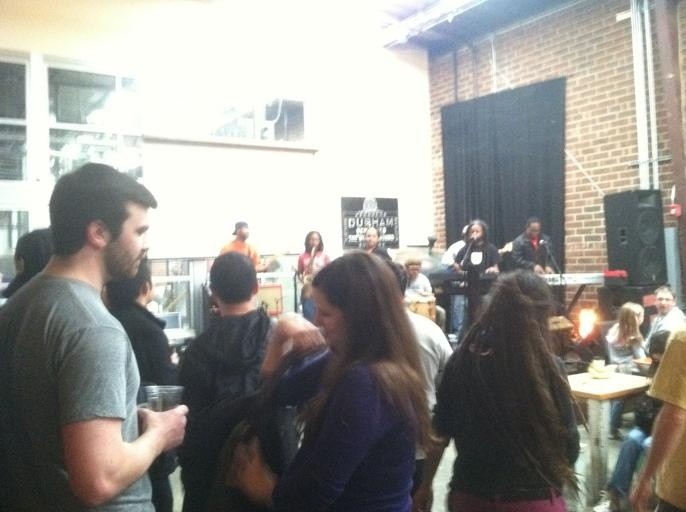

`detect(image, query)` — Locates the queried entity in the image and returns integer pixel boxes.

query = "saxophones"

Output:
[302,246,316,299]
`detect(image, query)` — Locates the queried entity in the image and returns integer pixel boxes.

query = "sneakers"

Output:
[593,490,621,512]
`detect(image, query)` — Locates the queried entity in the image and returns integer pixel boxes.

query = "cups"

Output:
[139,384,185,415]
[591,355,606,377]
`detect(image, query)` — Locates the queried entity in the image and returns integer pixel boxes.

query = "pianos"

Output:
[539,272,606,286]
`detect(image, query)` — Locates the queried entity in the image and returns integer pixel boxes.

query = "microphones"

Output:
[311,246,316,257]
[539,239,552,257]
[459,236,477,267]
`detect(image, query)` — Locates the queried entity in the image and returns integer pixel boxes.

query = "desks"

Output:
[566,370,656,505]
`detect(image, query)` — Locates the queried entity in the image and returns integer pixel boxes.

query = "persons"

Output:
[0,228,54,296]
[104,255,179,512]
[0,162,189,512]
[180,220,685,511]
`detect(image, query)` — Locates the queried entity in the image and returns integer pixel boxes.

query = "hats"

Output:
[232,222,248,234]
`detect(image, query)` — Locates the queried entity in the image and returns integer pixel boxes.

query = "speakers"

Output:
[603,190,667,285]
[266,99,304,141]
[599,285,672,414]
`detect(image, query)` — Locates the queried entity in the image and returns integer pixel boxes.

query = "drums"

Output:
[407,295,437,323]
